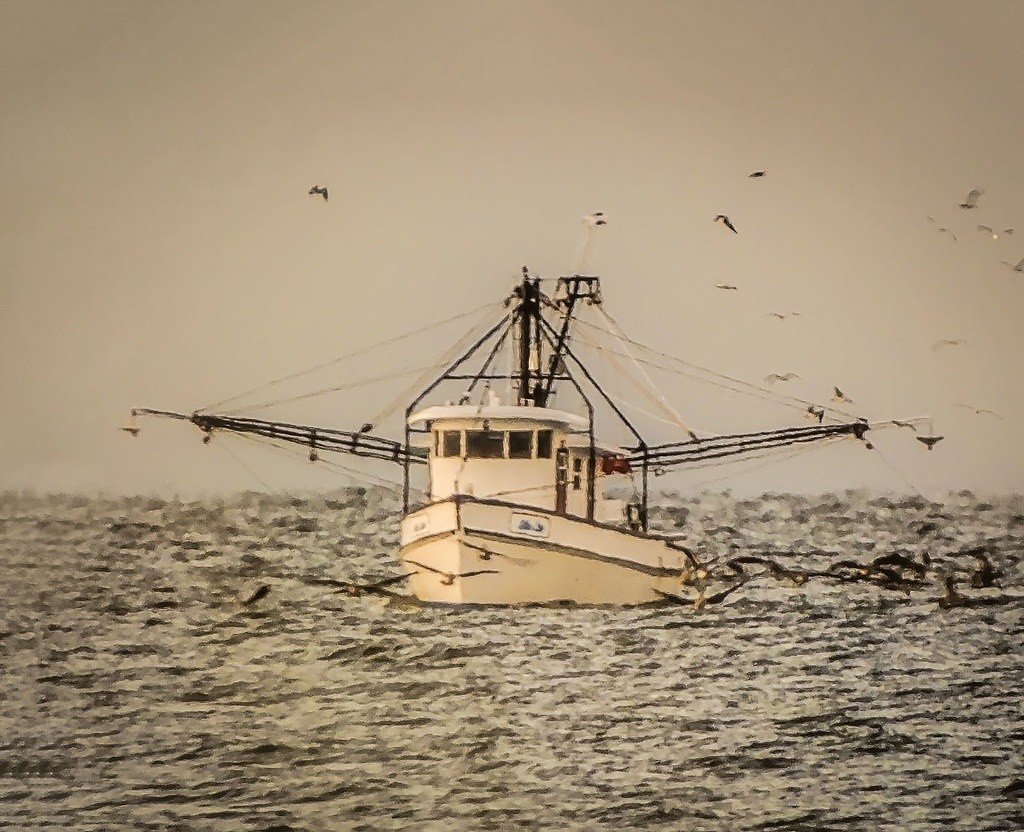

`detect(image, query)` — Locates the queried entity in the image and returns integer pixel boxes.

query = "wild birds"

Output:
[925,186,1023,275]
[767,311,801,321]
[712,212,738,236]
[231,582,273,610]
[650,531,1017,618]
[759,369,1001,460]
[747,169,767,179]
[926,337,971,351]
[308,185,329,203]
[714,284,737,291]
[118,426,142,439]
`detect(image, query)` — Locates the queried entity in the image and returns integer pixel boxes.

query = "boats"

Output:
[123,267,945,611]
